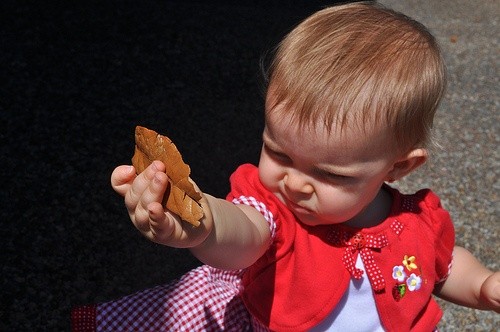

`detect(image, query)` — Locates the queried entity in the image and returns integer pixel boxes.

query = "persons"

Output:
[111,1,500,332]
